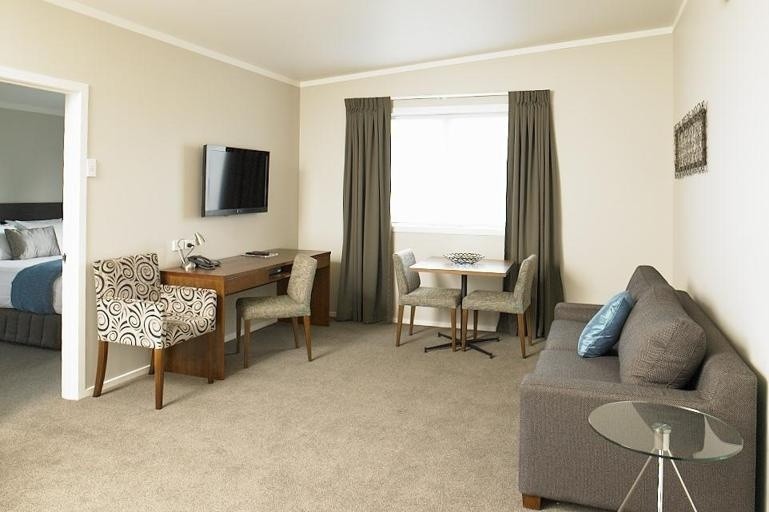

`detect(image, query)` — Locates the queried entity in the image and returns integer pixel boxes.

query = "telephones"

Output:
[187,256,215,270]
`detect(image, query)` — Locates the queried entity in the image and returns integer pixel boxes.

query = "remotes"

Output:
[245,250,269,256]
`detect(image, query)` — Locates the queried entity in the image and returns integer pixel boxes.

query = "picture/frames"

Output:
[672,100,707,176]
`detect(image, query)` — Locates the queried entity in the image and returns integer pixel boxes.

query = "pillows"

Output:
[577,290,633,358]
[0,218,63,260]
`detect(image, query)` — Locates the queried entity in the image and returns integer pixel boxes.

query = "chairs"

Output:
[236,253,318,368]
[91,250,219,410]
[392,247,536,360]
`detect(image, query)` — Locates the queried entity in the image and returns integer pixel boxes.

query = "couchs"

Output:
[518,265,758,511]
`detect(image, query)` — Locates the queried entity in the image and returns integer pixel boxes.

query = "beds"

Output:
[0,202,63,351]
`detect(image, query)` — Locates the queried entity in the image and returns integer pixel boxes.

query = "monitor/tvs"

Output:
[201,143,271,217]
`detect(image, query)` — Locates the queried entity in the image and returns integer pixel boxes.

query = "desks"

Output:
[155,248,332,380]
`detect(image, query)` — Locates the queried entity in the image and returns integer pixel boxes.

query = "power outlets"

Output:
[185,240,193,249]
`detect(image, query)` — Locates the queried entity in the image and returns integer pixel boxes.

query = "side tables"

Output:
[588,400,745,510]
